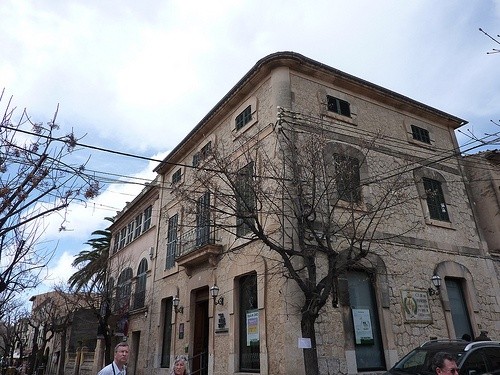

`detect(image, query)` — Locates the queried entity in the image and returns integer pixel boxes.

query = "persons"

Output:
[474,330,492,341]
[169,355,191,375]
[431,352,459,375]
[97,342,129,375]
[461,333,472,342]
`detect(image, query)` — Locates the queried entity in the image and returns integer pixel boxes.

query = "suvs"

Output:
[382,337,500,375]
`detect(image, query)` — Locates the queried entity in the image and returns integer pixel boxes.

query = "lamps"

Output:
[428,273,442,296]
[210,283,224,305]
[172,295,184,313]
